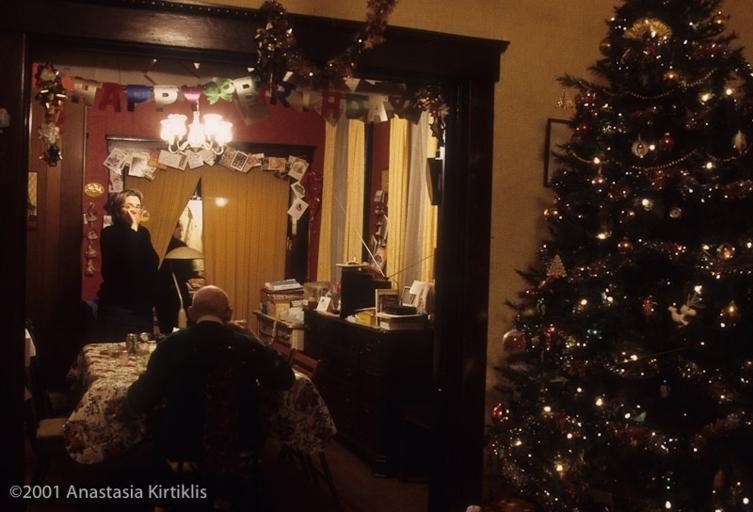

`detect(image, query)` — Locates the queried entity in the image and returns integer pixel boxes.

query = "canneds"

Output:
[125,334,136,353]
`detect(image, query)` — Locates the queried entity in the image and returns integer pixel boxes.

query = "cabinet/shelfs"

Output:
[277,320,303,350]
[251,311,276,344]
[302,298,432,469]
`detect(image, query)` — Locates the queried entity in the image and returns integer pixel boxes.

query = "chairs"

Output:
[254,348,342,505]
[23,388,72,495]
[269,335,295,359]
[25,327,72,419]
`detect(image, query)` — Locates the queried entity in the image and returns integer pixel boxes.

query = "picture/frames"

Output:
[543,118,574,185]
[375,288,400,327]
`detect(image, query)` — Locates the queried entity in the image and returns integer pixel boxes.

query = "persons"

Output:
[128,285,296,496]
[158,218,198,337]
[99,189,160,341]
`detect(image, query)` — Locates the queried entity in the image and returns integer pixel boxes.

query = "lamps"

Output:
[159,99,234,156]
[372,189,387,216]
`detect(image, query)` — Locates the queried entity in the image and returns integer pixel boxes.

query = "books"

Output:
[377,306,426,332]
[264,278,304,295]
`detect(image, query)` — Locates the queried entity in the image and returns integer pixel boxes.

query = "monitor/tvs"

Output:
[336,263,391,321]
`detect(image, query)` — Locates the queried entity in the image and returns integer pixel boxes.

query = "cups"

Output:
[138,210,150,221]
